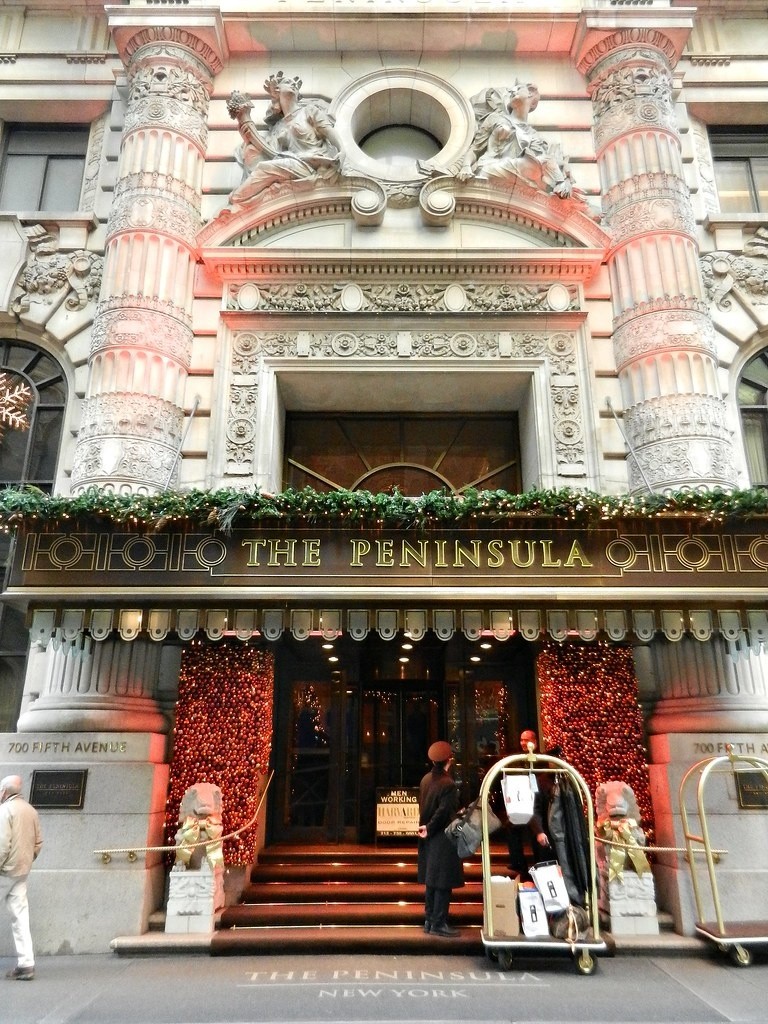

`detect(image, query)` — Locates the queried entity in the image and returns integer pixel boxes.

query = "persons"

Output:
[528,746,603,910]
[0,773,47,981]
[225,69,348,208]
[453,74,577,214]
[504,728,552,876]
[414,738,464,937]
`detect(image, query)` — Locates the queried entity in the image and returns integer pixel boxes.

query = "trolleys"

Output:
[480,742,768,976]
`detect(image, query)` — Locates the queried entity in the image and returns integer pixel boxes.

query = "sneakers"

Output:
[4,967,34,981]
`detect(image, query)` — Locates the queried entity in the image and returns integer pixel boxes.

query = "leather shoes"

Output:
[424,920,461,938]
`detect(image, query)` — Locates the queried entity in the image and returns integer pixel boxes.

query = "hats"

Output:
[428,741,452,762]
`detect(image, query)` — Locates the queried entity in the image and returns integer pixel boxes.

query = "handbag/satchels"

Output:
[445,797,502,858]
[500,768,539,825]
[491,860,590,955]
